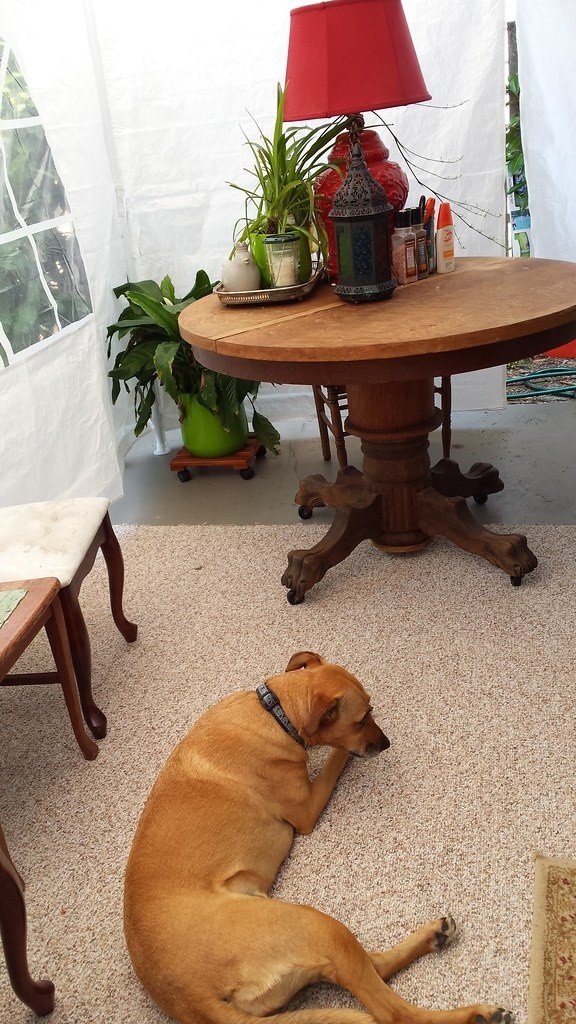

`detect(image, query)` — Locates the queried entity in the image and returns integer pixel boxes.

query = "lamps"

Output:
[326,141,399,304]
[280,0,432,306]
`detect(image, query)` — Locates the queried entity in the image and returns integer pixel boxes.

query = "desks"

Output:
[178,256,576,604]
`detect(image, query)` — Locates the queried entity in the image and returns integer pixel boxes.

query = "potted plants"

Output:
[228,82,395,289]
[105,269,282,457]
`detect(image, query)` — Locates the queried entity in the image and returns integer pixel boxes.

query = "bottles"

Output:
[391,209,417,284]
[406,207,429,280]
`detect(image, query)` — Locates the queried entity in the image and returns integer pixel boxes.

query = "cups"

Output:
[263,234,301,288]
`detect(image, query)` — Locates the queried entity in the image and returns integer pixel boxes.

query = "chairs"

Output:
[312,376,451,469]
[0,495,138,740]
[0,821,55,1019]
[0,577,99,761]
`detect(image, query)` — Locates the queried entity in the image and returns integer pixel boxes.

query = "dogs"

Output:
[123,650,518,1023]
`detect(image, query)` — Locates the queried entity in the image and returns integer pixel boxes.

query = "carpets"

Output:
[527,857,576,1024]
